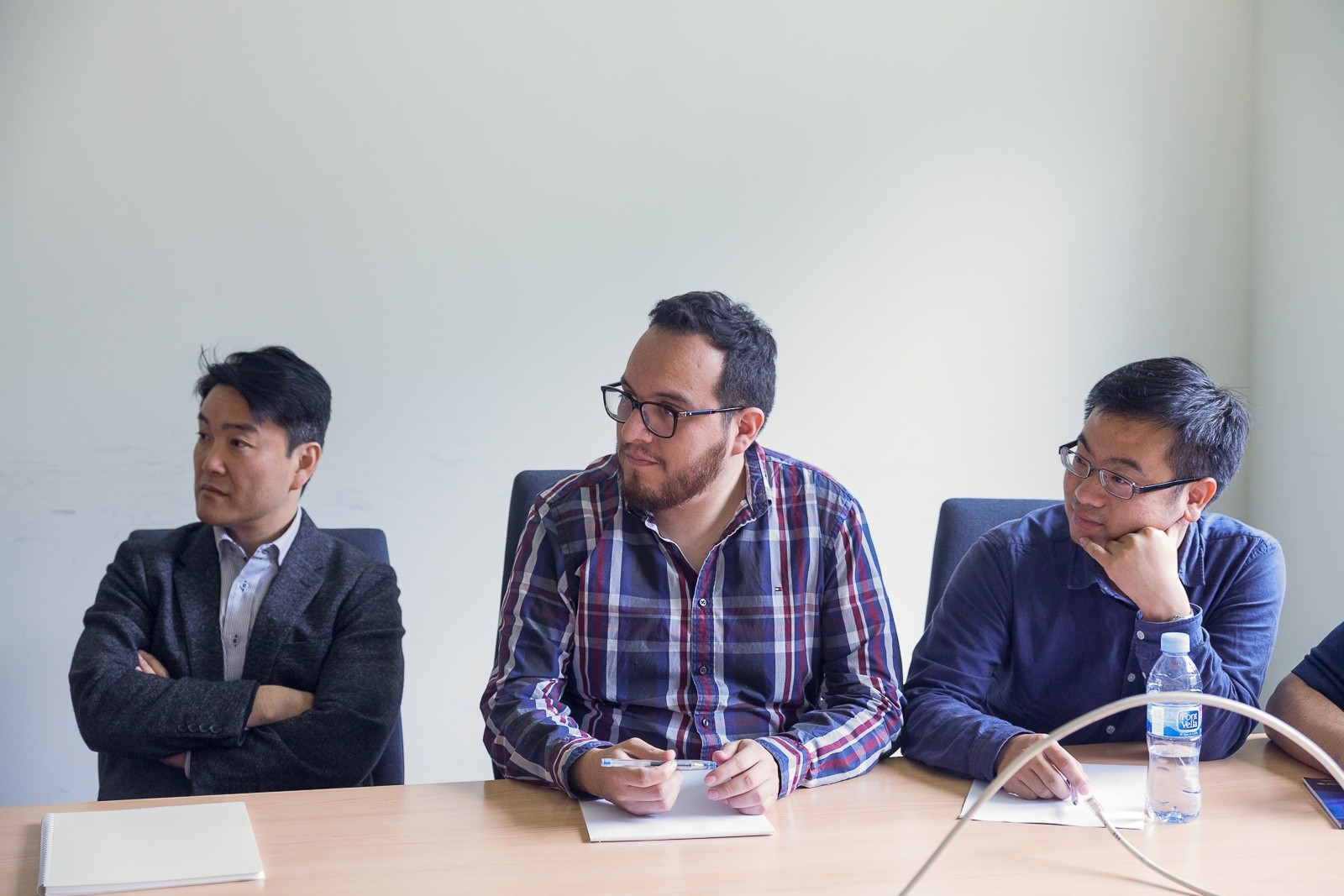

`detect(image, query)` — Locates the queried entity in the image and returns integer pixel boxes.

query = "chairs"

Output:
[926,498,1068,630]
[493,467,585,781]
[98,528,404,800]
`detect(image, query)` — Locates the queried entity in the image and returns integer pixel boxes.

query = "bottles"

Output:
[1146,631,1202,823]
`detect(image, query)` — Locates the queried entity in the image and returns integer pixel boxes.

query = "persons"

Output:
[66,344,405,797]
[479,291,905,815]
[900,356,1286,803]
[1262,619,1344,775]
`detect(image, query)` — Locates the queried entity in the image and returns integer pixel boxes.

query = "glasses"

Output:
[601,382,745,439]
[1058,440,1205,500]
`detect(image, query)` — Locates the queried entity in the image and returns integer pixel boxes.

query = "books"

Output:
[38,799,265,894]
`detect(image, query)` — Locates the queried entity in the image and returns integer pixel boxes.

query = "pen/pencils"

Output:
[1071,783,1078,805]
[600,758,717,770]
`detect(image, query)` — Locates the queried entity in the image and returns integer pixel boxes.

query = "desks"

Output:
[0,739,1344,896]
